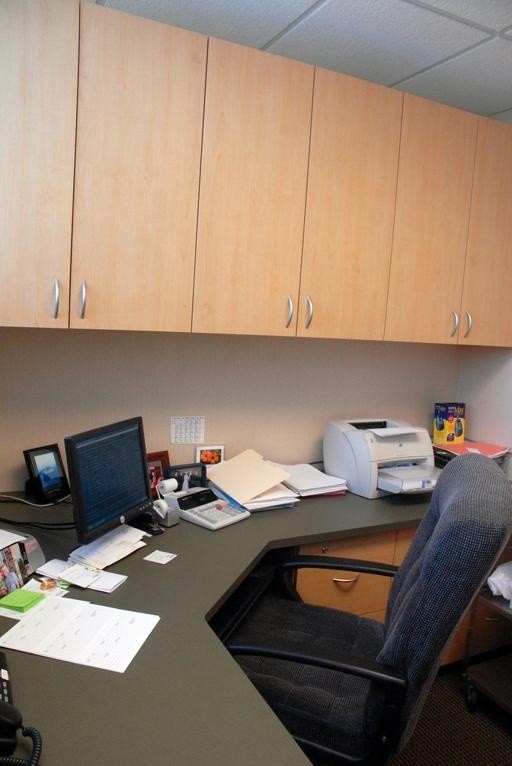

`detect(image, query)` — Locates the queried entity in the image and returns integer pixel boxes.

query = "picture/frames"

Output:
[22,444,67,501]
[146,446,226,498]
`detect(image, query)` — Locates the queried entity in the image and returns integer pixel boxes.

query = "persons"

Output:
[0,555,24,597]
[148,468,202,493]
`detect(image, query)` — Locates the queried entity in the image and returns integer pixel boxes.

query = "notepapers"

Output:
[0,588,46,613]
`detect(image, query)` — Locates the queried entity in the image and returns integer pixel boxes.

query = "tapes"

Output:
[152,499,168,514]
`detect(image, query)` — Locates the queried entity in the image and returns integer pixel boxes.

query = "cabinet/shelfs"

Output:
[385,89,509,348]
[0,0,206,335]
[190,37,404,341]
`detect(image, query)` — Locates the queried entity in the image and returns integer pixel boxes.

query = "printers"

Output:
[321,416,442,501]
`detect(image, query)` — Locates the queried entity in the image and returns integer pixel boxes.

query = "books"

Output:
[433,440,512,473]
[433,402,466,445]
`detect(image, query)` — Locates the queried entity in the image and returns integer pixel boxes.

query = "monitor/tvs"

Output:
[63,414,155,544]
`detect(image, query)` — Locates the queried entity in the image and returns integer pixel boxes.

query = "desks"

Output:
[9,439,503,763]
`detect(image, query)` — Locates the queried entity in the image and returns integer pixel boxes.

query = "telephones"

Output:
[0,651,23,755]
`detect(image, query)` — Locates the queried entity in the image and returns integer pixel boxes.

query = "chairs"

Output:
[208,453,504,763]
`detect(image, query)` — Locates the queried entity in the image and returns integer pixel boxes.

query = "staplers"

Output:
[143,511,163,536]
